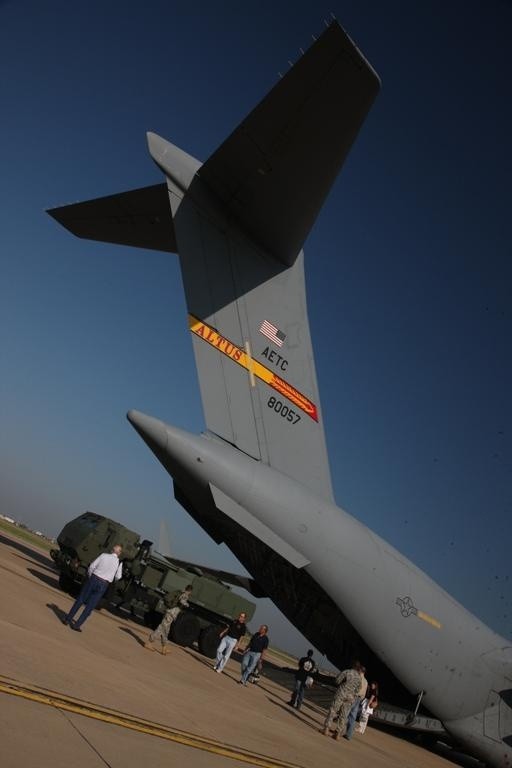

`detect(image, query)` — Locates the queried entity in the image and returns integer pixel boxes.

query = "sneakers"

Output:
[62,618,83,632]
[286,702,300,710]
[213,666,221,673]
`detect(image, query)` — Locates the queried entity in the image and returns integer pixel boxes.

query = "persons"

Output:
[236,624,269,686]
[212,612,247,673]
[62,543,124,632]
[317,661,363,742]
[352,680,379,735]
[285,650,316,709]
[143,584,195,654]
[329,665,369,740]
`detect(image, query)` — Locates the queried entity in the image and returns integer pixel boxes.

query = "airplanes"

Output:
[41,18,512,767]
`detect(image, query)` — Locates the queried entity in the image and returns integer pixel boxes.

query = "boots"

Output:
[144,639,154,650]
[161,646,172,655]
[319,728,350,742]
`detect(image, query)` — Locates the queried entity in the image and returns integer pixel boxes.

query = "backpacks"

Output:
[165,590,183,609]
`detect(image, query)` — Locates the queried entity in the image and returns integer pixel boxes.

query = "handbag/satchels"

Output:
[369,701,378,708]
[305,676,314,690]
[249,671,260,684]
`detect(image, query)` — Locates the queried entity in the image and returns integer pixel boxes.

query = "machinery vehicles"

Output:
[49,503,269,662]
